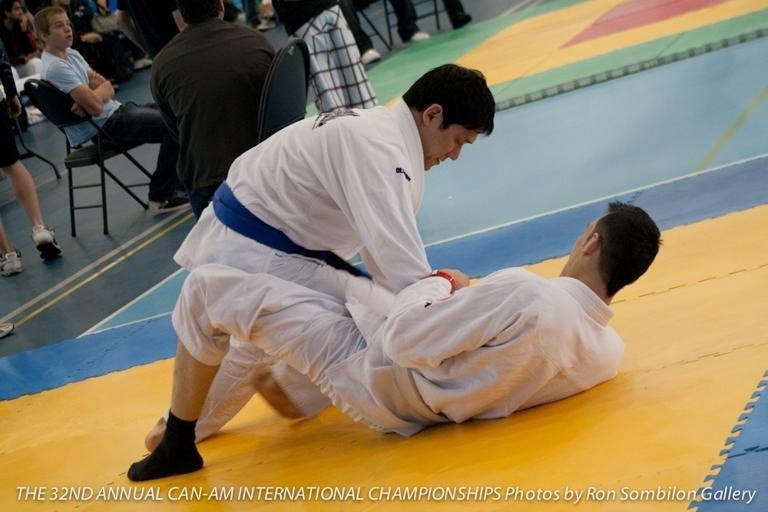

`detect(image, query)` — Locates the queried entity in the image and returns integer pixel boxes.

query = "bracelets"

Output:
[429,267,457,290]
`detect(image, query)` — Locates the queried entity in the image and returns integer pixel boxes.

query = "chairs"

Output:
[23,78,156,236]
[353,0,392,51]
[383,0,448,45]
[255,37,311,148]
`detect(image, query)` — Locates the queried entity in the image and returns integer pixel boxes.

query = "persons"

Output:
[170,63,495,420]
[128,199,661,482]
[0,0,469,275]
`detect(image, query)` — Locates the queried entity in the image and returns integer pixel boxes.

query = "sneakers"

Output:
[410,30,430,42]
[30,224,63,260]
[1,321,14,338]
[360,49,381,65]
[453,14,472,29]
[148,198,191,216]
[0,248,23,276]
